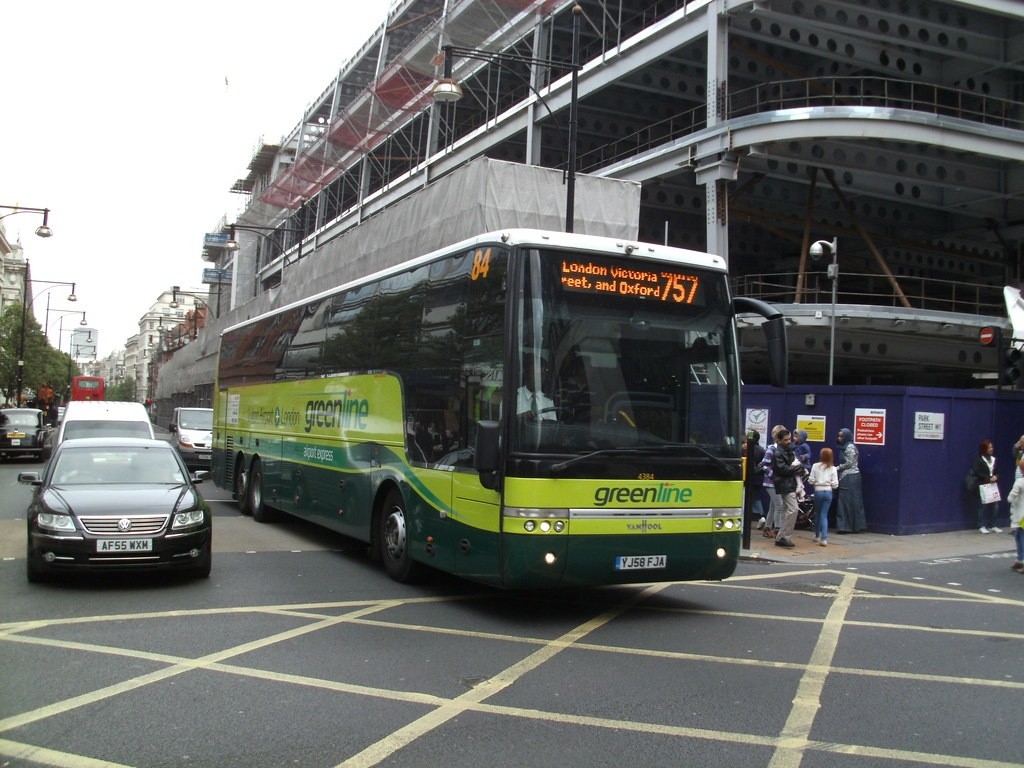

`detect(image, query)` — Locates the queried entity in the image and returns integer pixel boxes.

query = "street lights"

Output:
[221,197,308,265]
[170,270,222,320]
[148,302,198,362]
[428,5,583,234]
[17,257,77,409]
[59,315,97,405]
[809,237,837,385]
[43,292,88,348]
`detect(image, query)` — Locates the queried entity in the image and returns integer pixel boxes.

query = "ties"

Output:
[532,394,536,411]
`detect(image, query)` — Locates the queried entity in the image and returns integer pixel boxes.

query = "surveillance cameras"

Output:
[810,242,822,261]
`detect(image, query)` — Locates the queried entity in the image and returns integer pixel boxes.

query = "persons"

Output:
[416,347,463,435]
[557,355,601,422]
[760,425,804,546]
[808,448,839,546]
[27,382,58,427]
[498,354,578,479]
[742,429,765,529]
[791,429,811,502]
[1007,435,1024,569]
[835,427,867,533]
[974,439,1003,535]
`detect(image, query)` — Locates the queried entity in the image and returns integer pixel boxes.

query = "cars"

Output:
[18,436,213,584]
[0,408,54,464]
[57,406,66,423]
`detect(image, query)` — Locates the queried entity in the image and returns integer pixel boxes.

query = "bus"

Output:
[210,228,790,597]
[72,375,105,402]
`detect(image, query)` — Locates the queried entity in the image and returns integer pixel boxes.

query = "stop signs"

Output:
[979,326,997,347]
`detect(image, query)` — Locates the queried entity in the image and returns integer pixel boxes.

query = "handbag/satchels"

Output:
[979,482,1001,504]
[964,469,979,491]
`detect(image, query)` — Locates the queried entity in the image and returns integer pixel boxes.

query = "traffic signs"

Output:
[853,408,886,445]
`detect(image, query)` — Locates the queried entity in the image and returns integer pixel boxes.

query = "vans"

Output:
[49,400,154,461]
[168,407,213,474]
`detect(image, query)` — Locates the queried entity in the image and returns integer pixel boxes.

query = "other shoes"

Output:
[814,535,820,542]
[836,531,852,534]
[987,526,1003,533]
[976,526,989,533]
[799,490,806,499]
[775,538,795,547]
[757,516,765,530]
[795,484,804,494]
[774,528,779,534]
[820,540,827,545]
[1010,559,1024,573]
[763,528,778,538]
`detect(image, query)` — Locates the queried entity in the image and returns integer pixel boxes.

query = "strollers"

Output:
[795,492,818,532]
